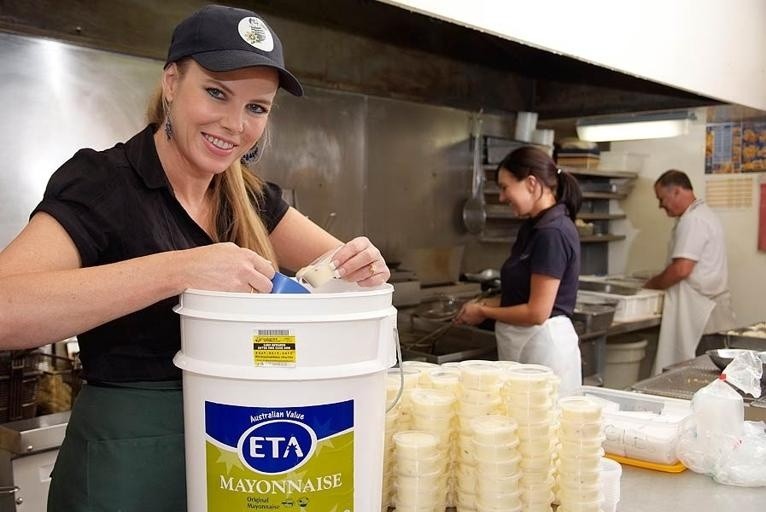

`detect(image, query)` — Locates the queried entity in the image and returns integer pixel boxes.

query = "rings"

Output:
[250,286,254,293]
[369,263,376,275]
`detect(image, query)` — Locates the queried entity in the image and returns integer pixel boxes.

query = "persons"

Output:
[0,2,393,511]
[640,168,736,378]
[453,146,584,387]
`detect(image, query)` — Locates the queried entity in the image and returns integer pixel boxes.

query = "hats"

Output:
[164,4,305,97]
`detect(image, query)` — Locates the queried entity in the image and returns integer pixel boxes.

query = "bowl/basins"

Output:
[706,348,766,371]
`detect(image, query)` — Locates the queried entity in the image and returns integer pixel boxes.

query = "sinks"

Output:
[395,316,496,367]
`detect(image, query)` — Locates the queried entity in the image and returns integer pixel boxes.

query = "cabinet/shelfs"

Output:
[474,165,637,243]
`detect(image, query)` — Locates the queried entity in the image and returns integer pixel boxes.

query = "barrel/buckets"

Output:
[172,288,404,512]
[602,332,650,391]
